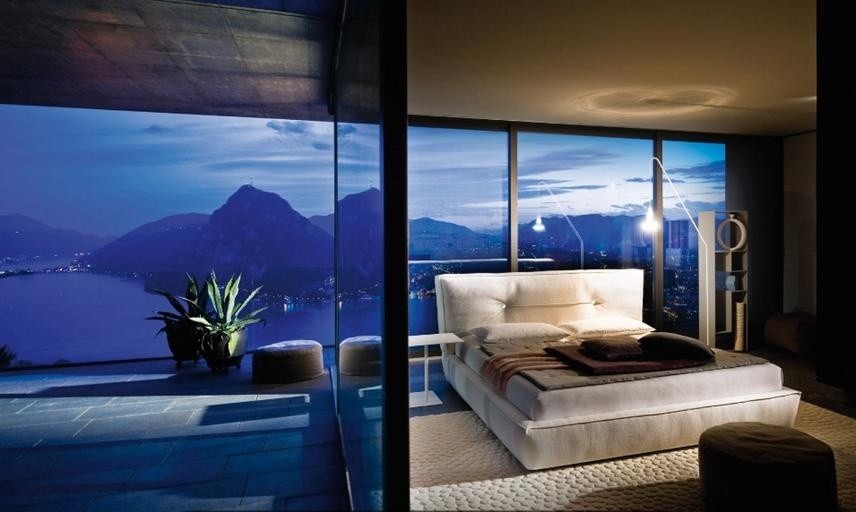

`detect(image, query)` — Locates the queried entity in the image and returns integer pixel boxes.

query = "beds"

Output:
[433,267,804,472]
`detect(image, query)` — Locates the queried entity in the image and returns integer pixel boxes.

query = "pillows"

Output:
[467,318,716,361]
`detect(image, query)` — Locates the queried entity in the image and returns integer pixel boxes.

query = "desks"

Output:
[409,332,465,401]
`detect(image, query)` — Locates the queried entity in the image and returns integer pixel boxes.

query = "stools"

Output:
[698,420,840,509]
[339,333,380,378]
[255,340,323,386]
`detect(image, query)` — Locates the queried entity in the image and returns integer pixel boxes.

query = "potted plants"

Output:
[145,270,212,362]
[174,271,268,372]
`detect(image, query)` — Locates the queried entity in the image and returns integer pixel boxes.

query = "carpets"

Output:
[373,401,854,512]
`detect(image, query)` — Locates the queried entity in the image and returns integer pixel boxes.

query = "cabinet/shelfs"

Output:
[696,210,753,350]
